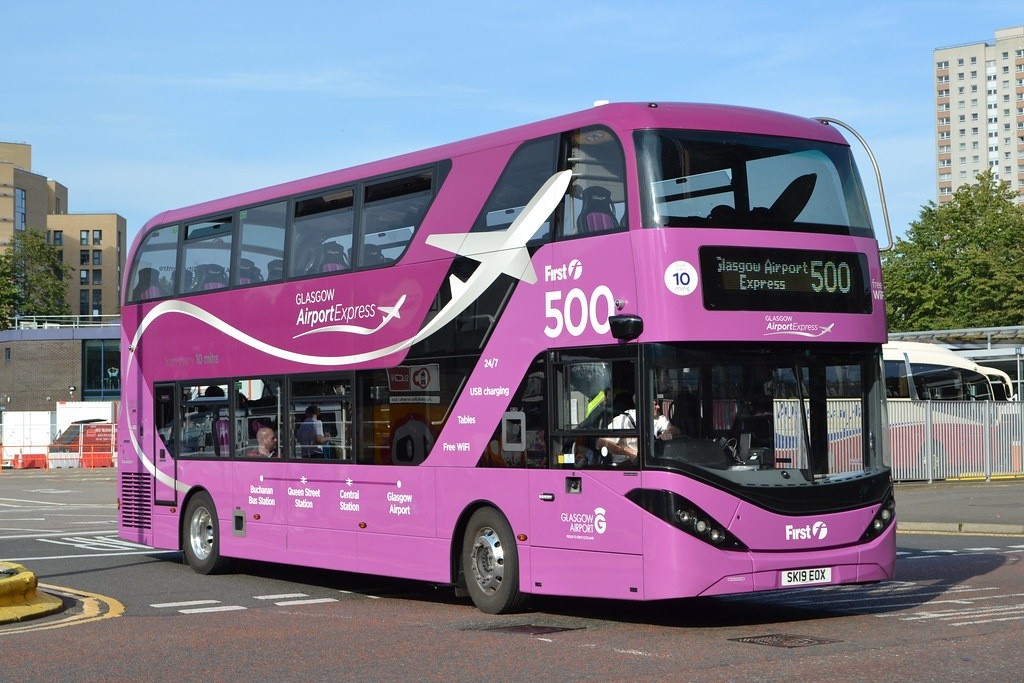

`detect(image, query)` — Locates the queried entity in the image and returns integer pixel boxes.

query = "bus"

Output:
[773,340,1014,481]
[116,100,902,617]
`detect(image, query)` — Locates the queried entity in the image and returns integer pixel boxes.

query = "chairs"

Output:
[605,393,632,465]
[576,186,622,234]
[135,241,397,300]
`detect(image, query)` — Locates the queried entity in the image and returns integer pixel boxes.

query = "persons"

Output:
[107,367,119,378]
[596,392,681,461]
[247,427,281,458]
[297,405,330,459]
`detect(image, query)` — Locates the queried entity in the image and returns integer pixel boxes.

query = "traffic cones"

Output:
[15,447,24,468]
